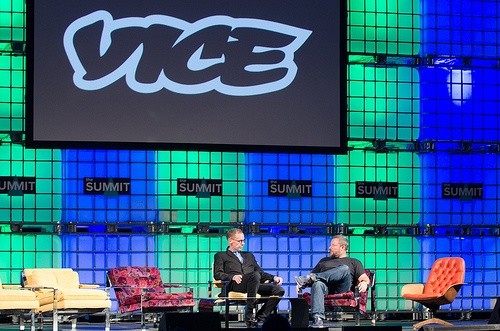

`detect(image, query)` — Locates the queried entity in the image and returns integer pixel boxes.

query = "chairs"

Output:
[105,267,196,331]
[20,268,111,331]
[0,280,43,331]
[302,269,376,325]
[400,257,467,331]
[208,263,278,329]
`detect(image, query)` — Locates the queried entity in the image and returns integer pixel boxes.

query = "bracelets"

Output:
[360,279,367,282]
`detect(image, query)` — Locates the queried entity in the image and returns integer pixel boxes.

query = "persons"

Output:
[295,235,371,328]
[213,227,285,329]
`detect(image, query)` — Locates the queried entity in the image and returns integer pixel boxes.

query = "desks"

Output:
[196,298,309,331]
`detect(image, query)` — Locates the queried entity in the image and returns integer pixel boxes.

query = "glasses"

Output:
[230,238,246,244]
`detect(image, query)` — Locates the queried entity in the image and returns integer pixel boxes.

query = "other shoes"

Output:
[255,316,264,325]
[310,317,324,328]
[244,306,255,324]
[294,274,313,290]
